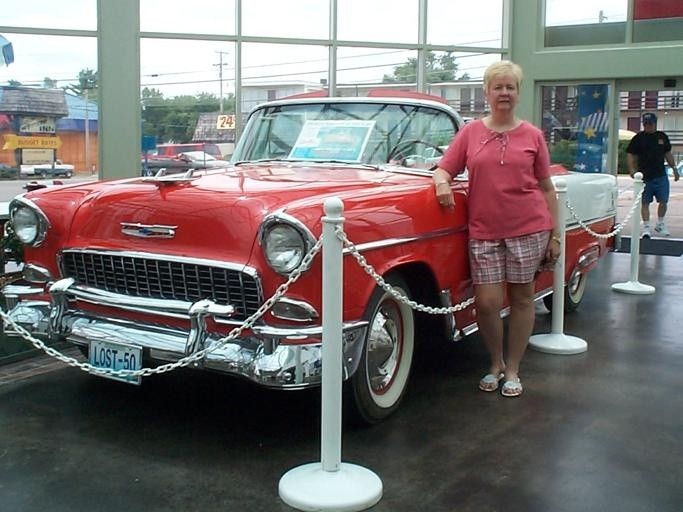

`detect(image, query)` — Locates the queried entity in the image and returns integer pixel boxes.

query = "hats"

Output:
[643,113,656,125]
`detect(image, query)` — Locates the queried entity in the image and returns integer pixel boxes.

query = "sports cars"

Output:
[140,150,229,177]
[1,90,618,426]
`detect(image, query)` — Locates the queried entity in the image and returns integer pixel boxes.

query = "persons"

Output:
[624,112,681,239]
[430,57,565,399]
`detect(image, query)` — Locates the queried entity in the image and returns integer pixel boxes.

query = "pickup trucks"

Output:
[19,160,75,177]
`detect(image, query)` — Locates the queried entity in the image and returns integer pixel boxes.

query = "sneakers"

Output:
[642,225,651,239]
[654,223,671,236]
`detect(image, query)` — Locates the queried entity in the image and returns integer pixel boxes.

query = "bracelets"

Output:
[434,180,449,188]
[550,237,561,245]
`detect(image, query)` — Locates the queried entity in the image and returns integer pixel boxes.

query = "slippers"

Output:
[478,373,524,396]
